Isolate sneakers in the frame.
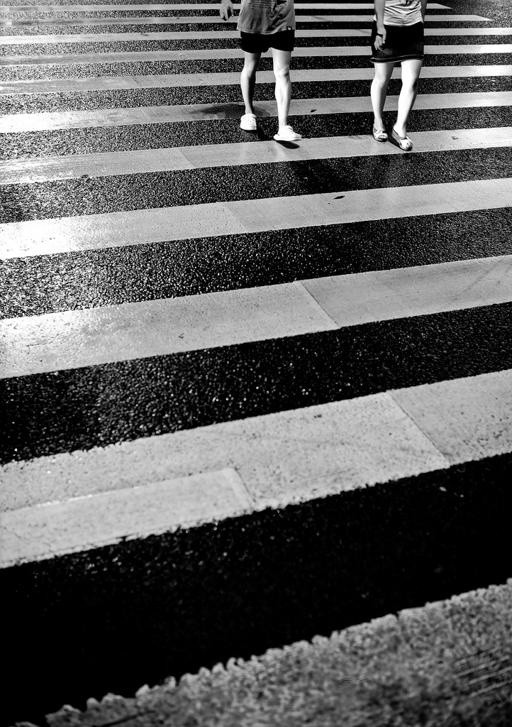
[392,129,413,150]
[373,127,389,141]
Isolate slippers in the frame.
[274,125,301,140]
[240,113,257,130]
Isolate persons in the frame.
[217,0,304,144]
[367,1,428,153]
